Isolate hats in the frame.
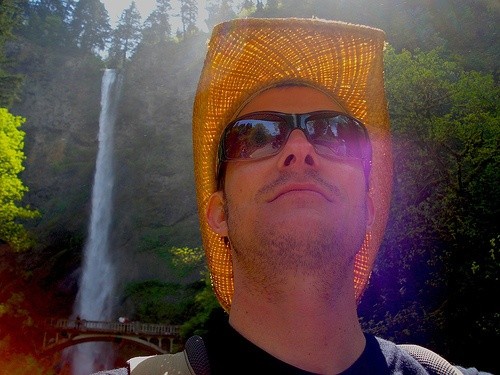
[192,17,393,314]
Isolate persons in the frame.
[89,80,492,375]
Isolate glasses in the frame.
[216,111,373,190]
